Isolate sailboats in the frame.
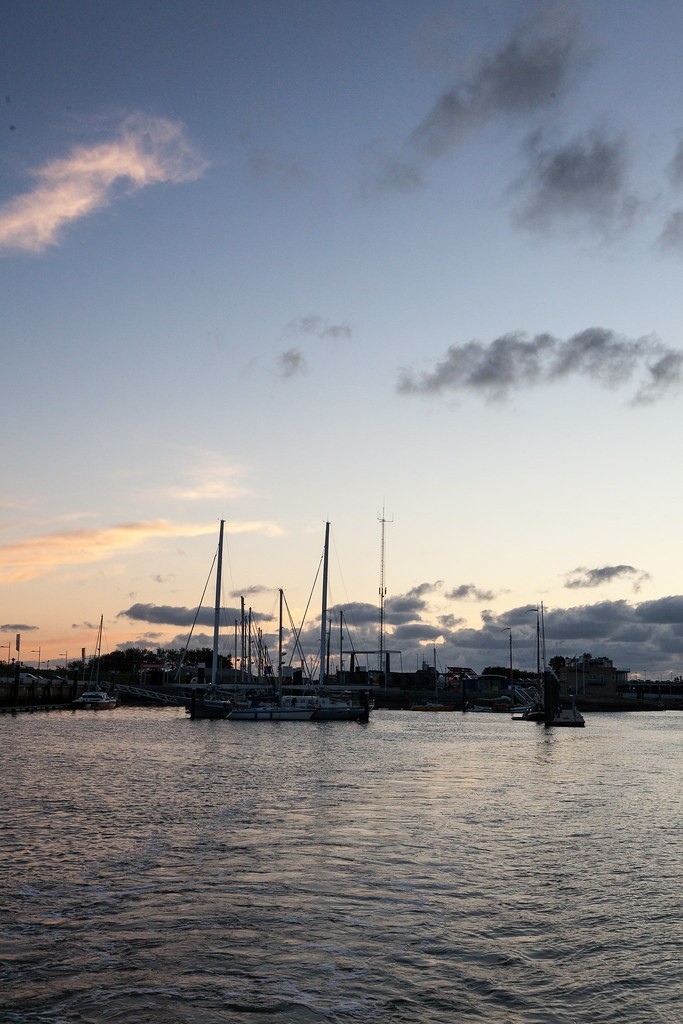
[70,614,117,710]
[170,520,389,722]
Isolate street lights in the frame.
[525,607,539,695]
[501,626,514,688]
[30,646,41,676]
[60,650,68,669]
[0,642,12,683]
